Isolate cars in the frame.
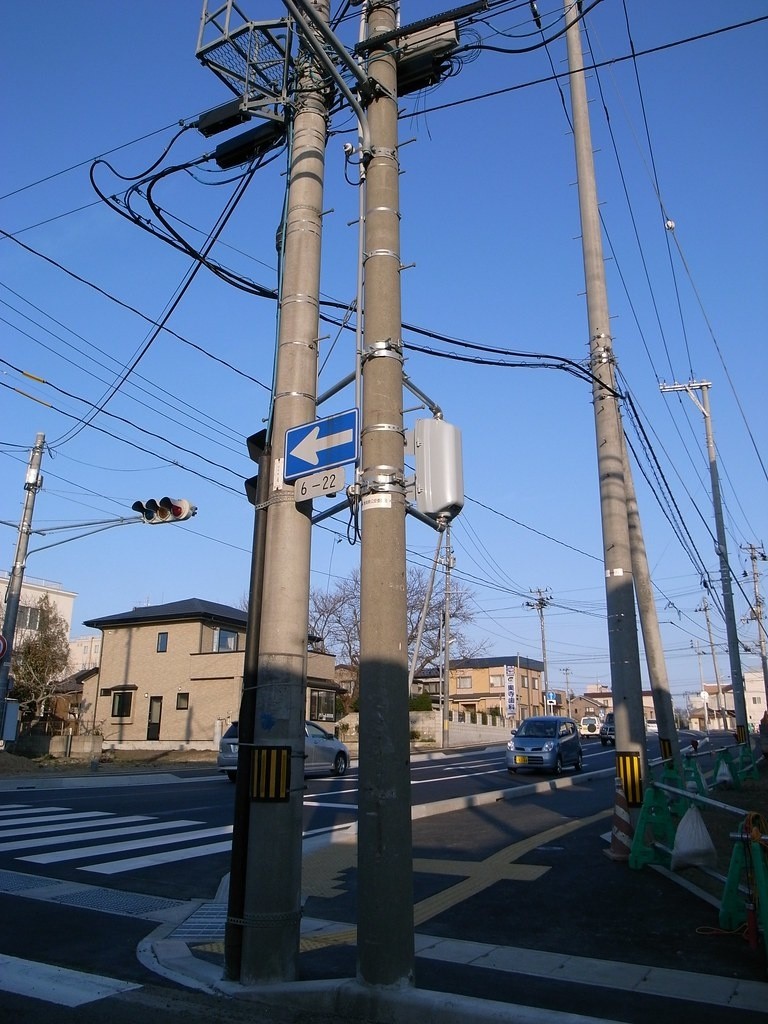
[214,716,351,786]
[504,715,584,777]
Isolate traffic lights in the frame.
[131,496,198,524]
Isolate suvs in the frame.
[600,712,615,746]
[578,716,604,739]
